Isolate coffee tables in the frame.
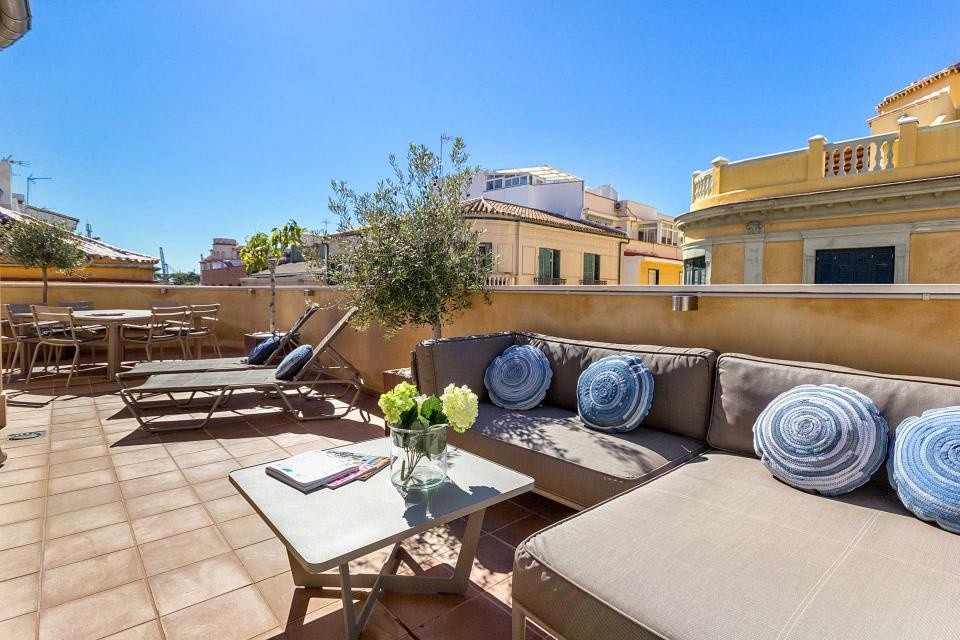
[229,434,536,640]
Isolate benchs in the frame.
[114,301,327,409]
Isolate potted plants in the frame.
[238,218,306,356]
[0,205,96,361]
[305,132,503,394]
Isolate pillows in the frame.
[751,383,890,499]
[886,405,960,535]
[577,353,655,433]
[484,344,554,412]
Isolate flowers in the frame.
[378,379,478,434]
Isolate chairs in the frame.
[119,303,371,434]
[0,299,224,396]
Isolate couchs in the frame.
[407,329,960,640]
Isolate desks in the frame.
[70,307,156,381]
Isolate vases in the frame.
[385,421,448,488]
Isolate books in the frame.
[265,449,391,493]
